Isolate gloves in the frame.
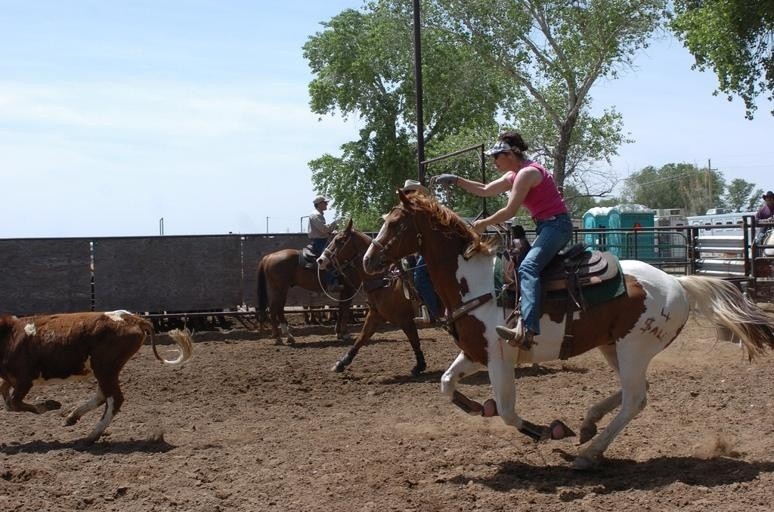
[430,174,458,184]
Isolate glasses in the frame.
[494,152,504,159]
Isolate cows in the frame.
[1,309,193,444]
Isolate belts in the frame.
[533,216,556,222]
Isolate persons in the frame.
[306,195,345,293]
[395,177,442,328]
[428,132,575,349]
[753,191,774,257]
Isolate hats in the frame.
[396,179,429,194]
[313,196,330,204]
[483,140,521,156]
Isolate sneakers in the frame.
[327,284,345,292]
[496,326,534,350]
[413,316,439,324]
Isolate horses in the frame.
[363,187,774,472]
[314,219,446,375]
[254,248,357,346]
